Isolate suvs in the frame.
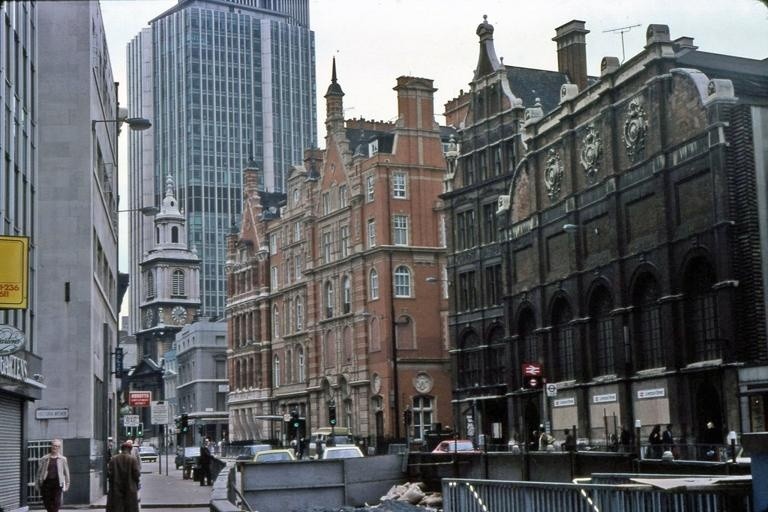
[236,445,272,460]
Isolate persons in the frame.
[562,428,576,451]
[609,432,618,452]
[292,434,335,460]
[200,438,214,487]
[508,426,547,451]
[707,423,738,447]
[105,440,144,512]
[649,423,675,456]
[38,439,70,512]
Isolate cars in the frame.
[138,446,157,463]
[316,444,365,460]
[254,449,295,462]
[176,447,201,469]
[431,438,483,454]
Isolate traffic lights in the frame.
[174,417,181,429]
[329,406,337,427]
[127,426,131,438]
[294,421,300,429]
[182,414,189,433]
[521,363,543,390]
[137,423,143,435]
[292,409,297,419]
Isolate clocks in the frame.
[171,306,188,323]
